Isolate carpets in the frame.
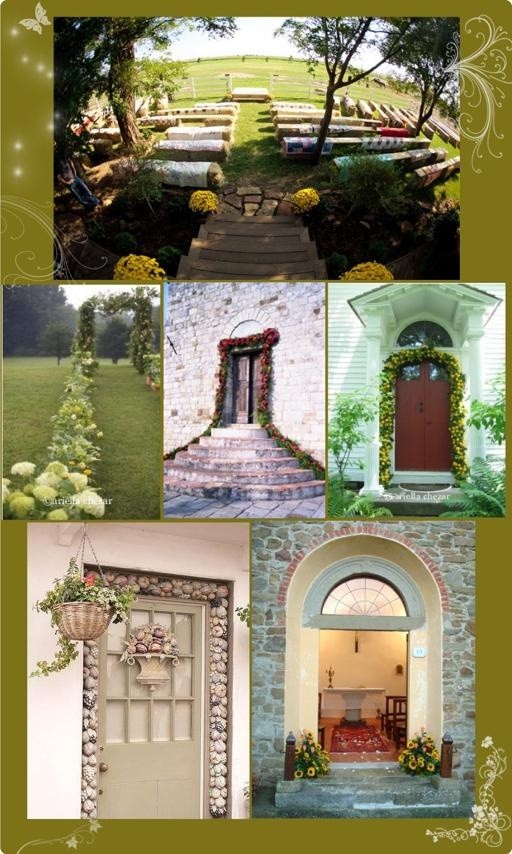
[328,723,391,753]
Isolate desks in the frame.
[322,686,386,728]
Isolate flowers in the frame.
[287,186,321,215]
[376,335,473,490]
[293,727,332,781]
[28,555,140,679]
[188,189,219,217]
[163,325,327,482]
[2,283,161,519]
[222,91,233,98]
[336,259,394,280]
[395,725,442,779]
[111,251,169,280]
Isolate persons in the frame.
[57,159,100,209]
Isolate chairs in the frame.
[318,692,327,750]
[380,694,407,752]
